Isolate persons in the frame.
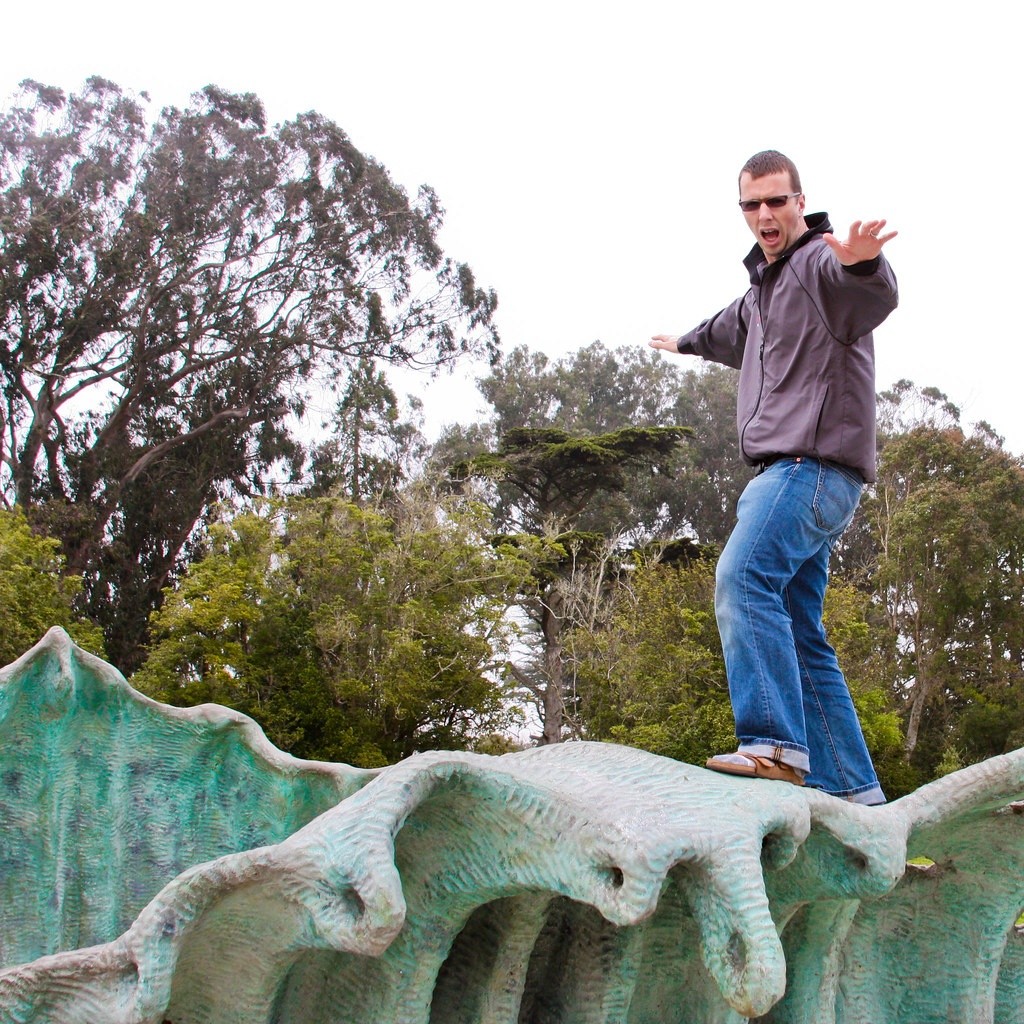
[648,150,900,806]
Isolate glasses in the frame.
[738,192,802,212]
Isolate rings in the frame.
[870,231,878,237]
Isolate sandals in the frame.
[705,752,805,785]
